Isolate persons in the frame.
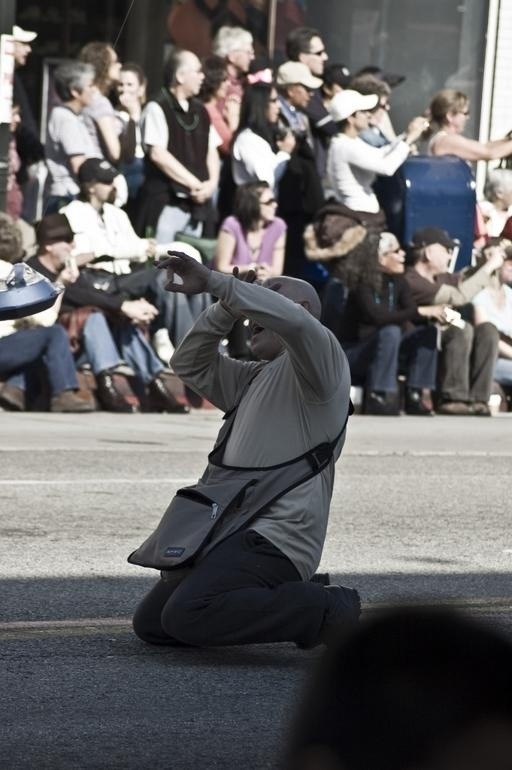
[0,23,512,417]
[133,249,360,653]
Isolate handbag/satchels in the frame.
[128,455,302,571]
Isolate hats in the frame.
[32,214,77,246]
[13,26,38,43]
[325,89,379,123]
[325,62,352,87]
[413,224,458,250]
[277,60,324,91]
[361,66,407,87]
[76,158,121,184]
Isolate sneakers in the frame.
[1,371,192,414]
[368,389,491,415]
[297,573,362,647]
